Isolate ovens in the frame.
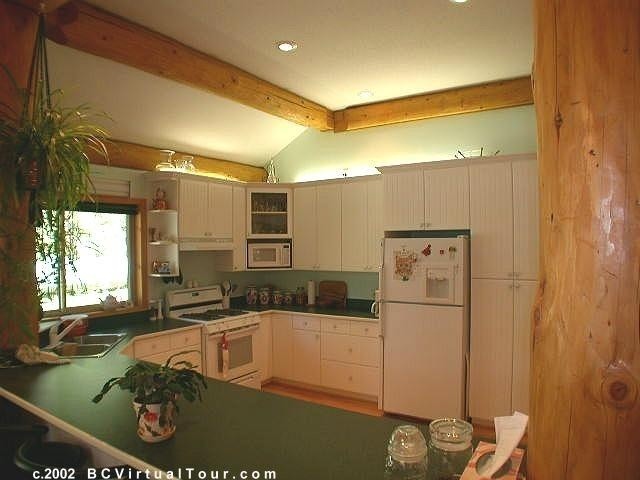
[204,314,262,384]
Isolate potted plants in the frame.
[0,13,121,364]
[91,349,207,443]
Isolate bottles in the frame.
[382,425,430,480]
[428,416,475,480]
[271,290,295,306]
[258,287,270,305]
[245,285,258,305]
[295,286,306,307]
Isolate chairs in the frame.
[0,422,148,479]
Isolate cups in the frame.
[222,296,230,308]
[120,300,126,305]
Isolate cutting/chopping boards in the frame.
[319,280,347,304]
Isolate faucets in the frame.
[49,316,84,343]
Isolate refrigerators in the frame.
[377,234,470,423]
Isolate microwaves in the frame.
[248,241,292,269]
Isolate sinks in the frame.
[65,333,127,345]
[45,343,111,358]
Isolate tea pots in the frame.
[98,294,117,305]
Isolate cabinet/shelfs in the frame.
[142,175,180,279]
[133,328,202,377]
[272,314,379,404]
[291,179,382,271]
[239,187,291,239]
[382,166,469,231]
[178,178,238,251]
[465,161,542,422]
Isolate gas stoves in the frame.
[177,307,250,322]
